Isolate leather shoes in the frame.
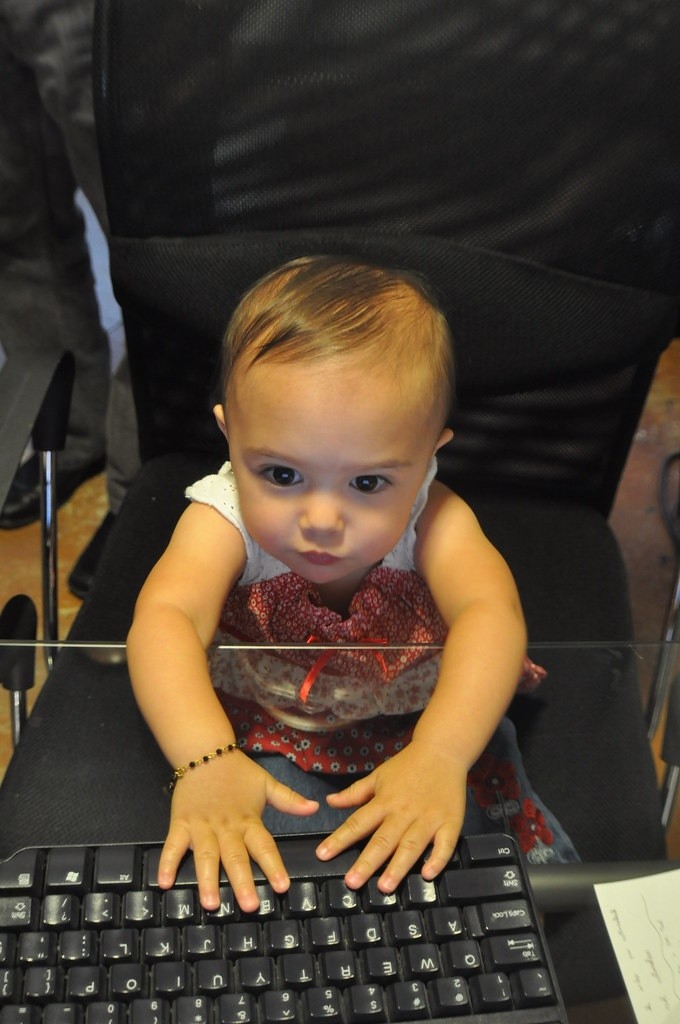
[0,455,105,529]
[68,511,115,599]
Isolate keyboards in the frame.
[0,832,571,1024]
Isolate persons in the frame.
[126,255,582,911]
[0,0,145,601]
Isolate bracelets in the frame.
[163,740,240,800]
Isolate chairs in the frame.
[0,0,680,861]
